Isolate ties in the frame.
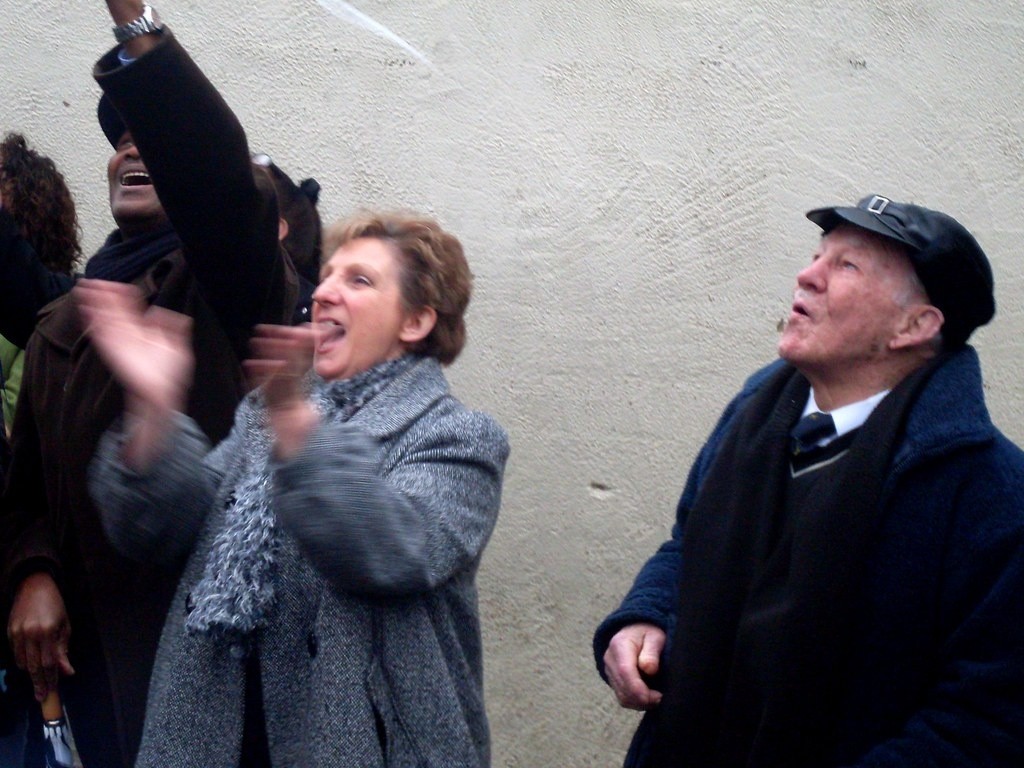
[790,412,835,457]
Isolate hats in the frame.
[97,92,131,153]
[806,193,995,360]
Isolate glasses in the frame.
[250,153,283,180]
[115,137,135,151]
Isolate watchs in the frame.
[111,5,163,45]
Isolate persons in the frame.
[0,0,327,768]
[593,198,1024,768]
[71,214,509,768]
